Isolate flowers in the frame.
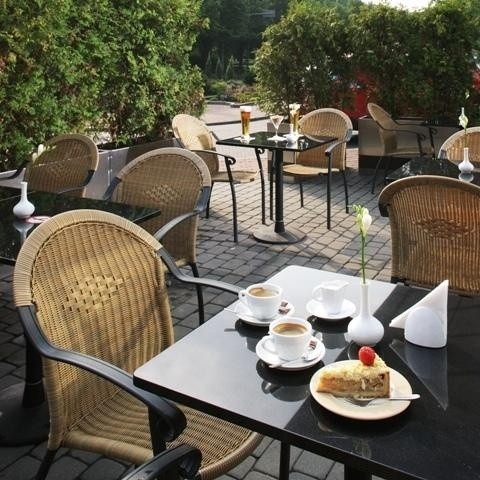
[344,198,375,284]
[288,102,301,124]
[25,133,58,181]
[454,104,471,147]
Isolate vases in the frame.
[11,217,35,248]
[11,180,37,219]
[346,283,385,348]
[459,147,476,174]
[458,171,475,183]
[286,123,299,144]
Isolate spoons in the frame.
[269,350,321,367]
[223,307,281,322]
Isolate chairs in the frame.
[119,437,205,480]
[364,99,443,196]
[166,110,269,243]
[99,144,216,333]
[0,130,97,201]
[7,203,294,480]
[373,171,480,298]
[436,126,479,161]
[266,105,356,230]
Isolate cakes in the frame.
[317,348,390,400]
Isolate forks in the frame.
[353,393,420,403]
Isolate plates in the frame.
[309,360,412,420]
[256,335,325,371]
[306,298,357,321]
[233,297,294,327]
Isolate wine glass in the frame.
[271,115,284,140]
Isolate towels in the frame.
[388,335,451,413]
[385,278,452,330]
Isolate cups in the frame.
[261,317,312,361]
[238,283,283,320]
[239,106,253,139]
[312,280,352,315]
